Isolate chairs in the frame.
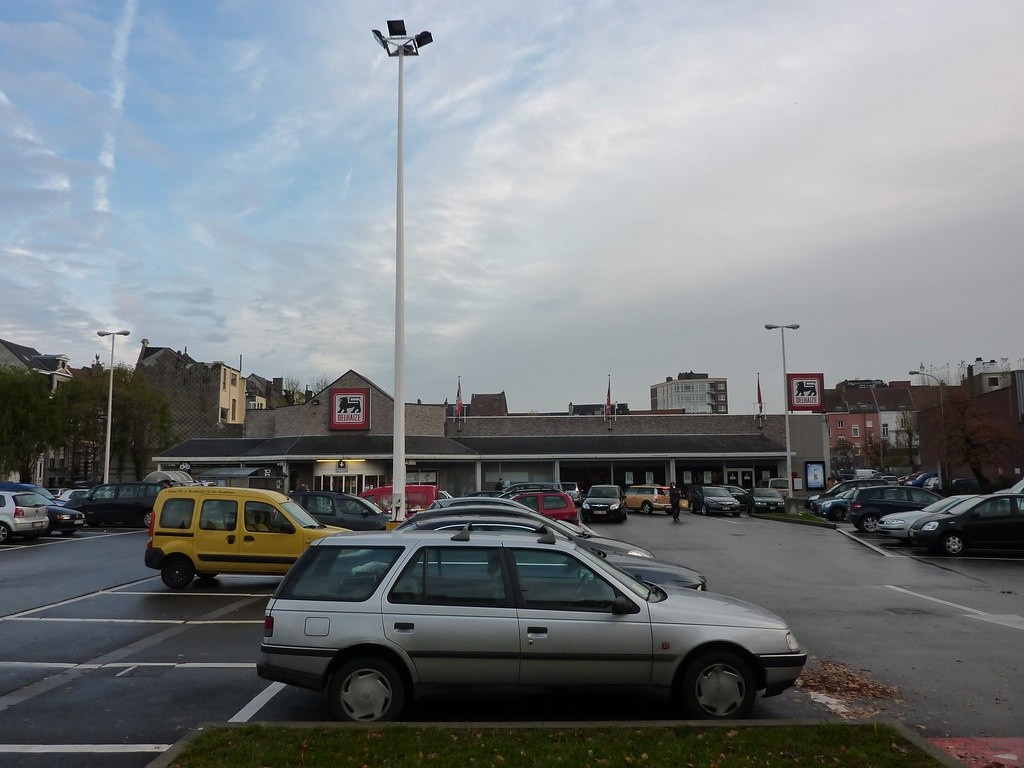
[246,511,269,532]
[120,487,131,499]
[986,501,999,515]
[310,497,319,512]
[486,557,525,605]
[893,490,906,500]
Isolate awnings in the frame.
[197,467,264,479]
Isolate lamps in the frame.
[312,399,320,404]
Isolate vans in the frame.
[63,483,163,528]
[806,479,946,534]
[145,484,439,589]
[758,479,790,498]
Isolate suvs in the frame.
[460,482,670,524]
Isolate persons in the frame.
[301,483,310,510]
[826,477,832,489]
[833,478,837,485]
[156,480,174,498]
[745,487,756,514]
[669,482,681,522]
[263,504,295,533]
[495,478,503,495]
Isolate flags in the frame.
[604,377,612,414]
[455,380,463,416]
[758,374,763,412]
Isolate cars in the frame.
[876,495,1024,556]
[688,485,785,517]
[0,483,85,545]
[257,497,806,721]
[880,471,980,494]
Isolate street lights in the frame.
[764,323,801,497]
[908,371,948,479]
[97,329,130,483]
[373,18,433,530]
